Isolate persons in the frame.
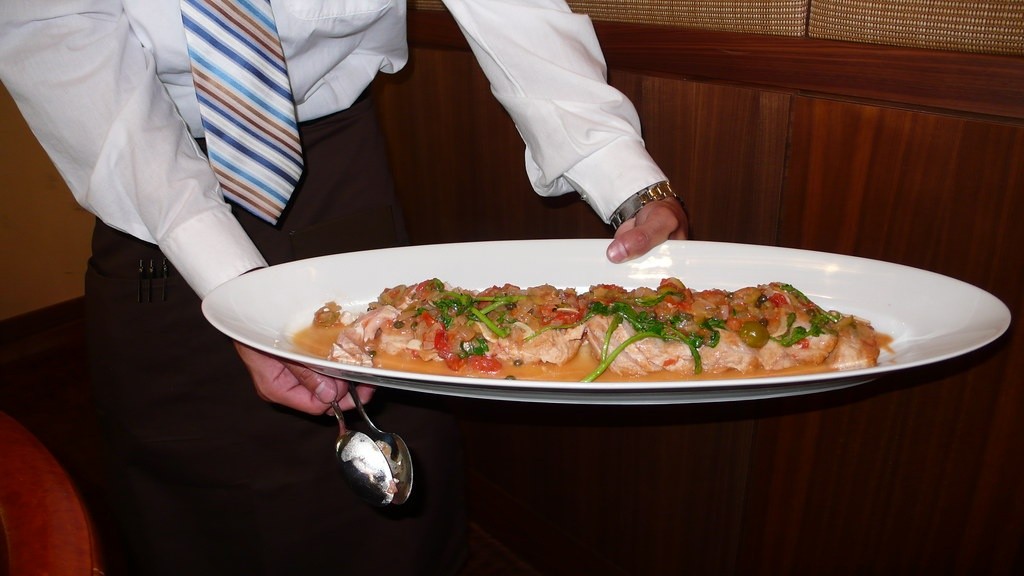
[0,0,687,576]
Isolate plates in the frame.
[201,237,1014,408]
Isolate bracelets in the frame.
[609,180,687,231]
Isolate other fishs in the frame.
[312,282,878,371]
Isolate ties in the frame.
[180,0,304,224]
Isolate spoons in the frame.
[329,400,395,507]
[344,383,414,509]
